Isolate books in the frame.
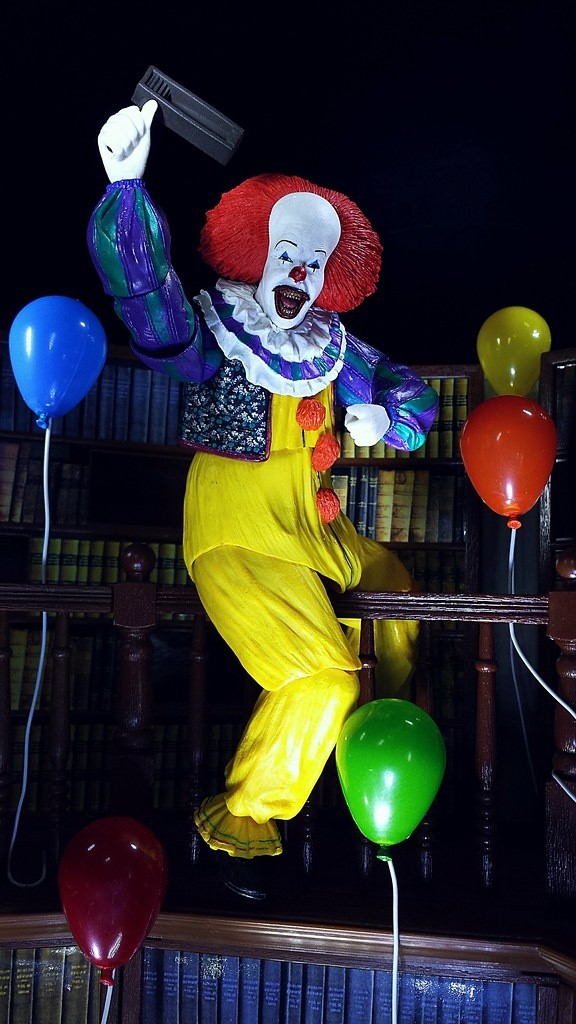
[0,443,465,543]
[25,537,210,621]
[9,628,244,813]
[432,665,457,735]
[0,350,469,459]
[0,943,575,1023]
[387,549,467,630]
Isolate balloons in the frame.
[475,304,553,400]
[59,816,161,985]
[10,295,107,426]
[334,700,441,860]
[460,396,557,527]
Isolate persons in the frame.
[85,100,440,921]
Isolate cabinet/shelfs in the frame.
[0,286,572,736]
